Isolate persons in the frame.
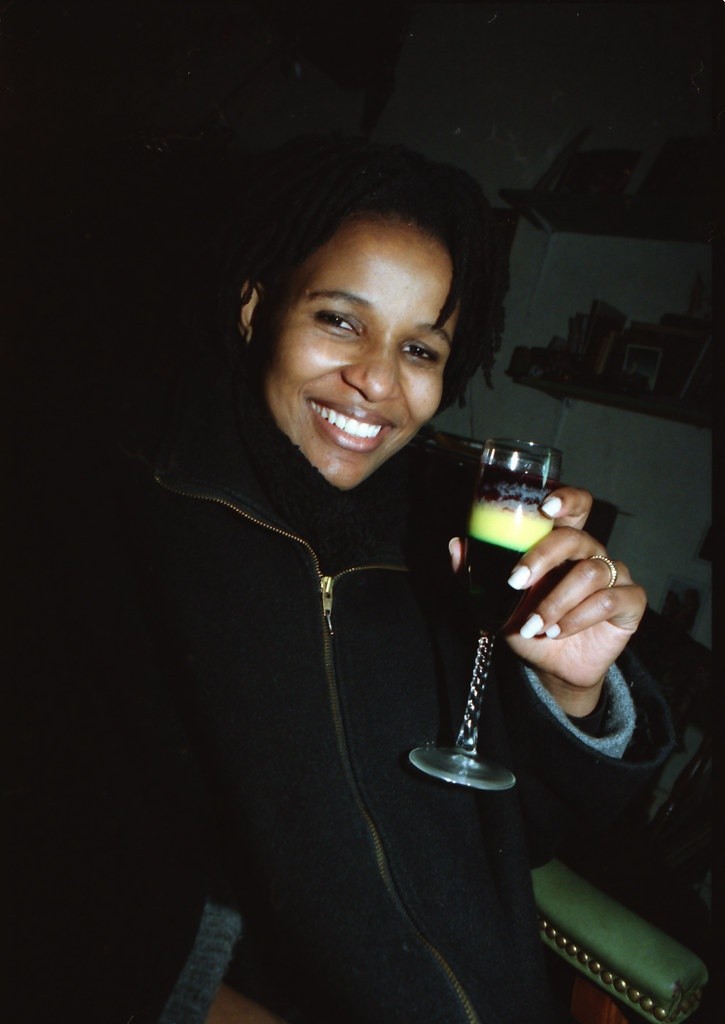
[0,143,646,1024]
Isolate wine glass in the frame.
[410,438,563,791]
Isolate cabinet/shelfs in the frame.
[498,184,713,428]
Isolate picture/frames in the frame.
[693,520,713,567]
[614,336,671,403]
[656,573,711,640]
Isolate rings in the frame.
[590,555,618,587]
